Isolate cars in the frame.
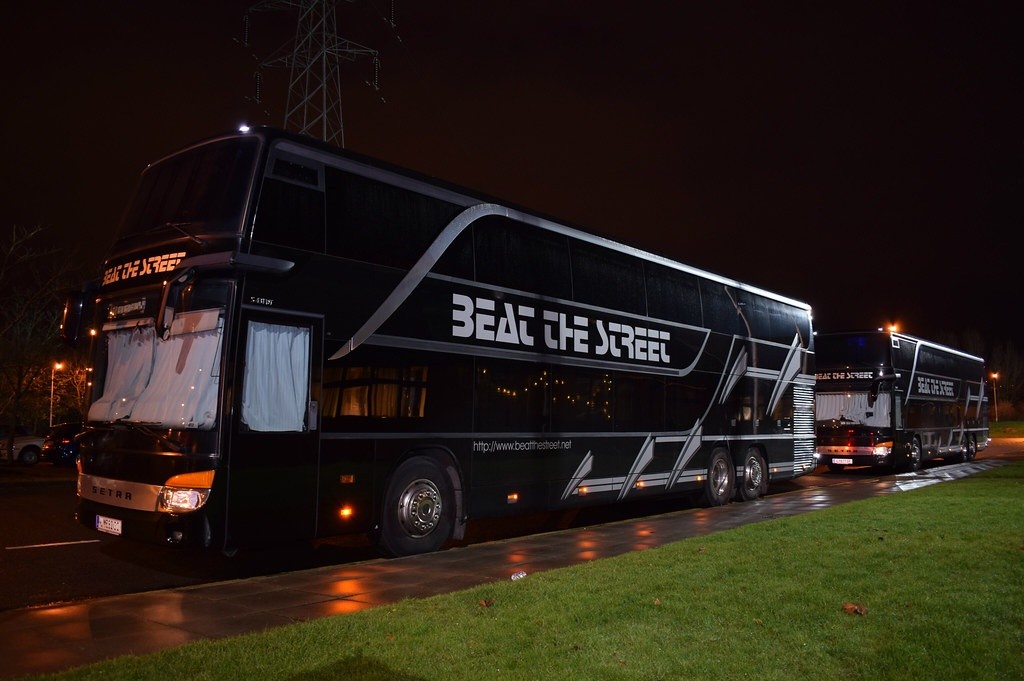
[39,418,84,474]
[0,433,49,467]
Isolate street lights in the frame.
[990,373,999,423]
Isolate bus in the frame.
[812,326,991,473]
[59,123,818,559]
